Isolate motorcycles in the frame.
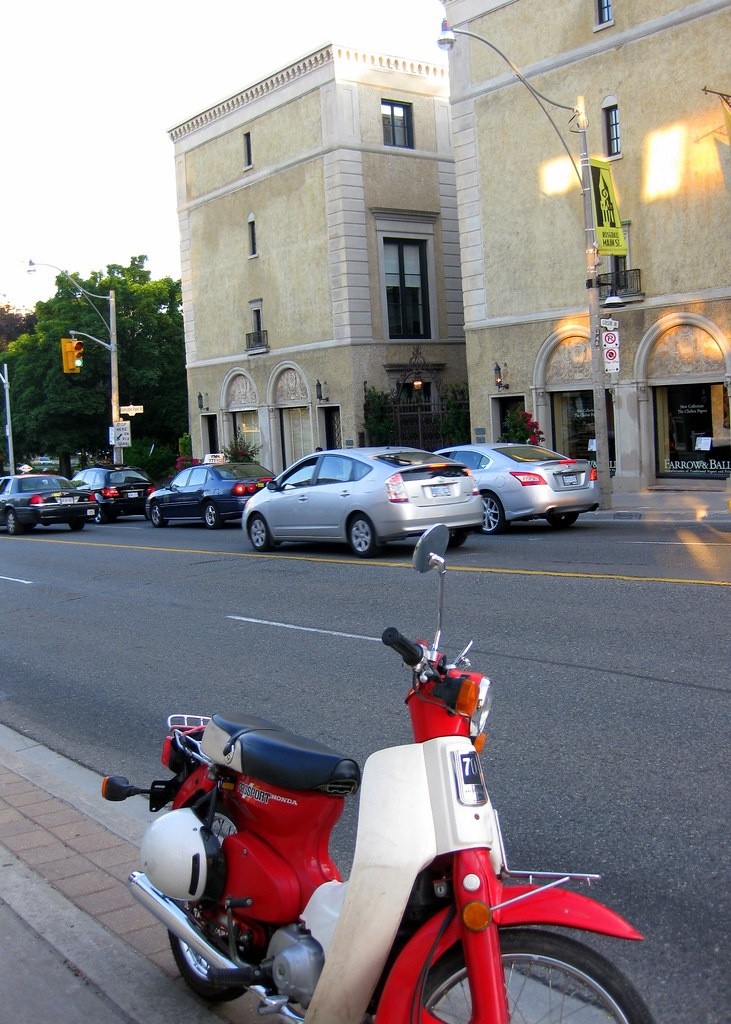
[100,521,660,1023]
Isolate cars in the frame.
[241,443,484,558]
[33,457,54,465]
[69,464,156,525]
[0,474,101,535]
[432,442,602,536]
[143,453,278,530]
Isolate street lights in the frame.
[436,20,618,508]
[391,370,424,446]
[26,259,126,465]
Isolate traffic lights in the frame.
[72,340,84,367]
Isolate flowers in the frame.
[174,457,198,470]
[499,402,546,446]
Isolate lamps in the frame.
[494,362,509,391]
[389,345,430,401]
